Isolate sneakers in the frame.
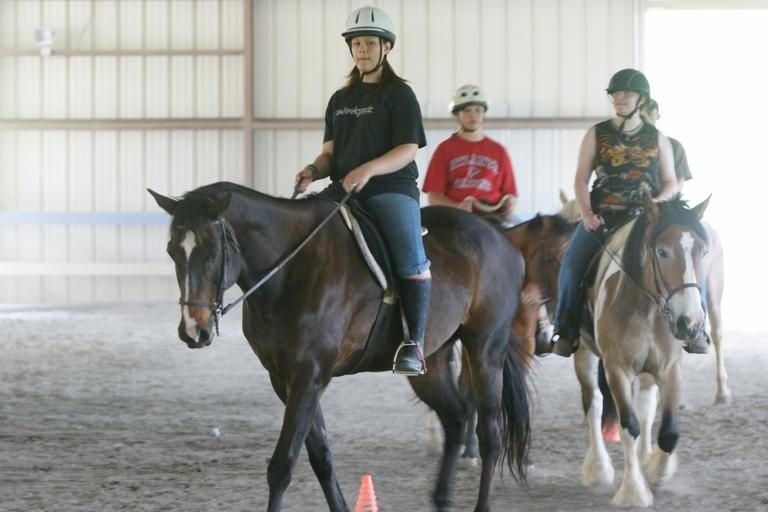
[552,337,575,357]
[686,334,710,355]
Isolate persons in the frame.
[640,98,693,198]
[423,85,518,223]
[294,7,432,373]
[551,69,712,357]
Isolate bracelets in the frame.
[306,164,319,180]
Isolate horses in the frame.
[569,187,714,509]
[454,208,574,467]
[553,186,731,444]
[144,181,536,512]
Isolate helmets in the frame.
[606,69,650,99]
[341,7,397,43]
[451,83,489,111]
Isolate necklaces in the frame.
[624,122,642,133]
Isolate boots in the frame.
[396,279,431,373]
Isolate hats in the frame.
[644,98,658,110]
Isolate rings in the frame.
[351,181,357,186]
